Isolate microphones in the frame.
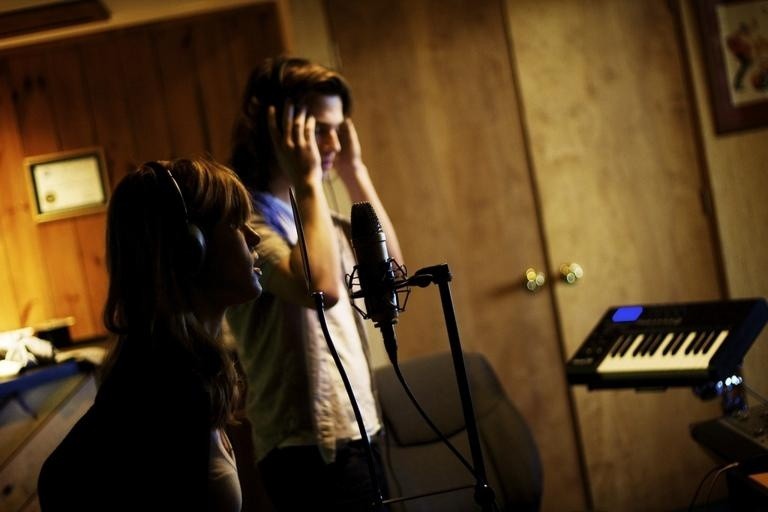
[350,199,400,325]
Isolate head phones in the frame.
[148,161,207,272]
[257,59,301,139]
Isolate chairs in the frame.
[370,348,545,511]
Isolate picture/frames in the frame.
[22,144,112,226]
[695,1,768,136]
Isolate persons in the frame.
[226,56,406,511]
[38,158,263,510]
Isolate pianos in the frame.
[564,299,768,389]
